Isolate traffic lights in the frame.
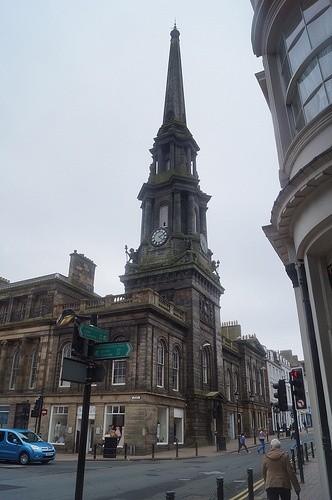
[60,356,88,386]
[272,378,288,412]
[291,367,308,409]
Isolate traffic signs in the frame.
[91,341,133,360]
[78,323,109,343]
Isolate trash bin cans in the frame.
[217,435,228,452]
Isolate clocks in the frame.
[200,233,208,255]
[150,228,170,247]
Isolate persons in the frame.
[107,427,117,436]
[238,434,251,454]
[9,435,17,441]
[263,439,300,500]
[39,432,48,442]
[55,421,64,443]
[27,435,34,440]
[292,429,295,438]
[258,429,266,453]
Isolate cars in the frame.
[0,428,56,464]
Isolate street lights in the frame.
[55,309,100,500]
